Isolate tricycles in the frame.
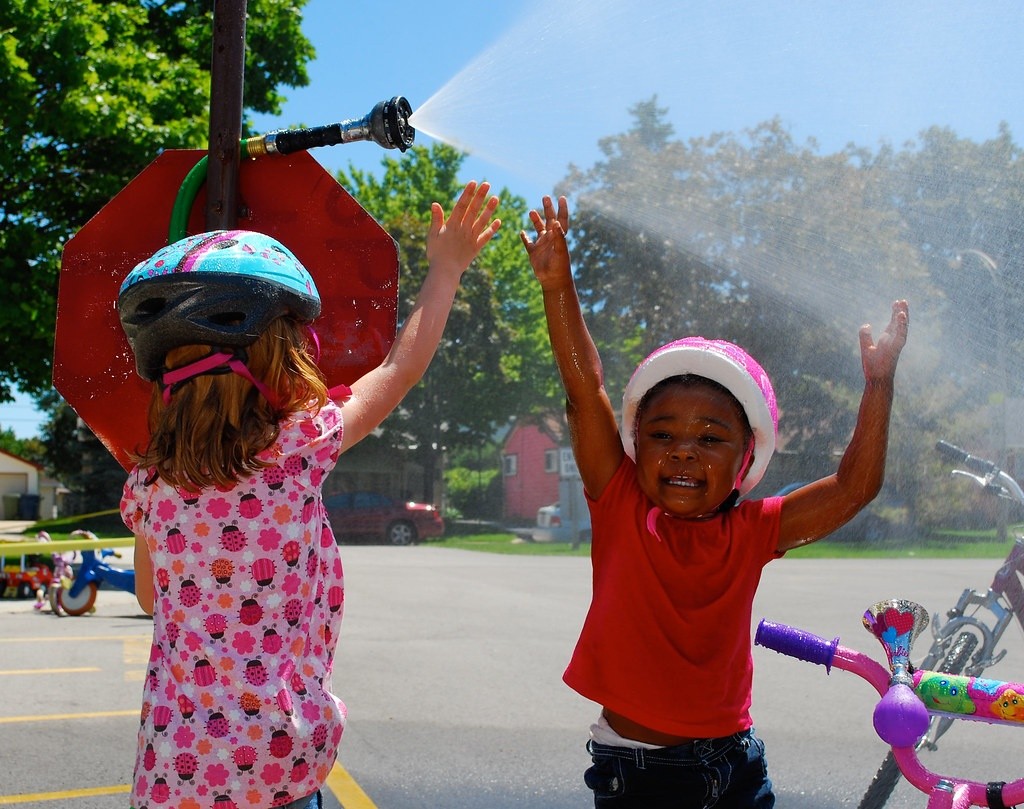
[39,528,144,616]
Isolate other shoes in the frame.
[34,602,44,610]
[57,604,63,610]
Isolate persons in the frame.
[33,560,52,611]
[37,529,52,541]
[117,178,502,809]
[53,556,74,581]
[79,526,98,539]
[521,194,910,809]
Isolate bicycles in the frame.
[754,595,1024,809]
[856,435,1024,808]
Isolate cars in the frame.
[0,538,53,599]
[534,502,562,531]
[322,487,445,545]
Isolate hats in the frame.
[53,556,66,565]
[622,335,780,501]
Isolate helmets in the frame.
[119,229,323,384]
